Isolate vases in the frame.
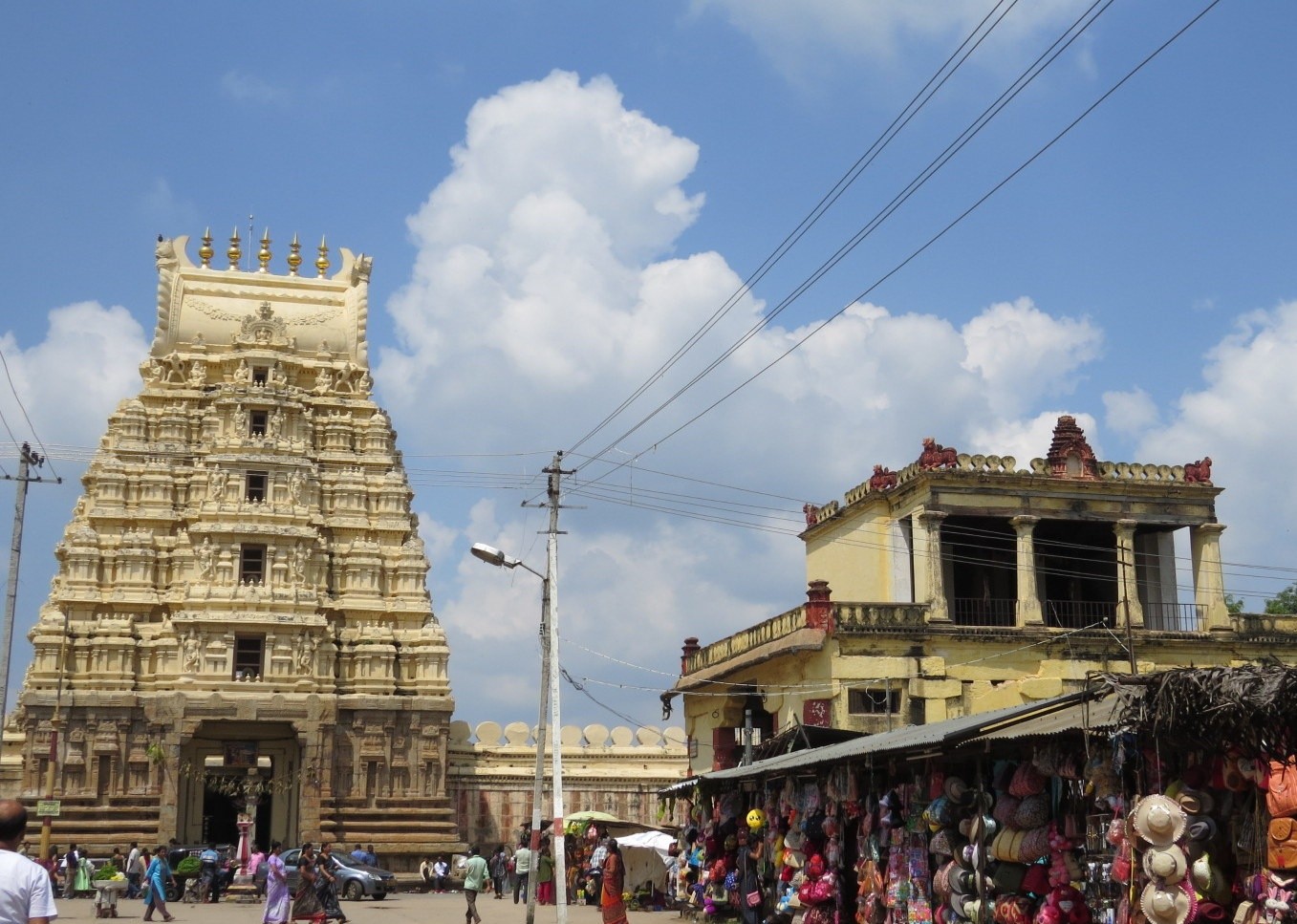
[92,880,129,918]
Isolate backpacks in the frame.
[1264,747,1297,817]
[1267,818,1297,871]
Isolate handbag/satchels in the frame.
[781,797,809,924]
[746,891,761,907]
[668,827,704,907]
[927,760,959,924]
[991,738,1079,924]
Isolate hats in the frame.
[1123,779,1231,924]
[942,775,998,924]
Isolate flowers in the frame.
[92,865,129,882]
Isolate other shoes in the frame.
[597,908,602,911]
[440,891,443,894]
[165,916,175,922]
[465,913,471,924]
[435,891,438,894]
[474,918,481,924]
[494,893,502,899]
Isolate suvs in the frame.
[160,843,238,903]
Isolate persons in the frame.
[0,799,58,924]
[352,843,380,869]
[736,832,764,924]
[18,838,223,922]
[249,841,349,924]
[460,845,492,923]
[488,832,629,924]
[420,856,452,894]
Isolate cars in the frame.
[252,847,397,901]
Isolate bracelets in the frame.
[328,875,331,879]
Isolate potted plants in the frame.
[577,889,586,906]
[621,892,632,908]
[177,856,203,903]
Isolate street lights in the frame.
[467,539,551,924]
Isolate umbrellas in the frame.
[564,809,616,821]
[613,830,679,896]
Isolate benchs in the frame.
[394,872,466,892]
